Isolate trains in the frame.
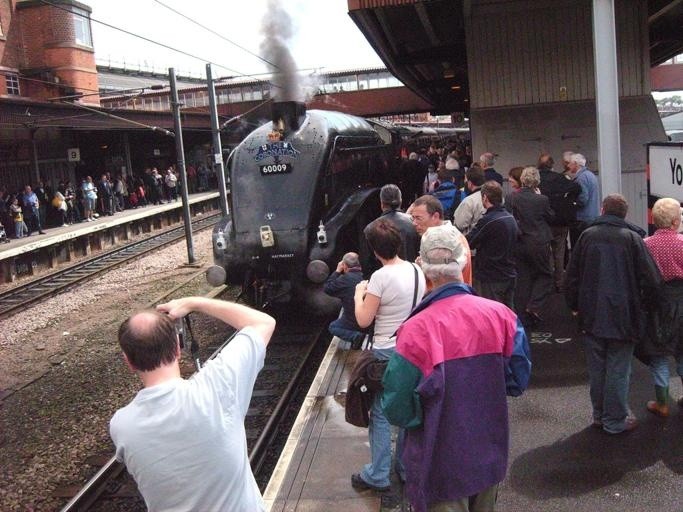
[206,97,471,311]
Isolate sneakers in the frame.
[591,412,638,434]
[351,331,366,350]
[16,193,180,240]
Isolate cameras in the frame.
[162,312,186,349]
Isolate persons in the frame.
[0,159,217,246]
[106,292,279,512]
[322,134,681,509]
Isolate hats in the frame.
[419,224,465,266]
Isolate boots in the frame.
[646,384,670,418]
[678,375,683,406]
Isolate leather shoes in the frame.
[525,306,545,322]
[350,470,392,493]
